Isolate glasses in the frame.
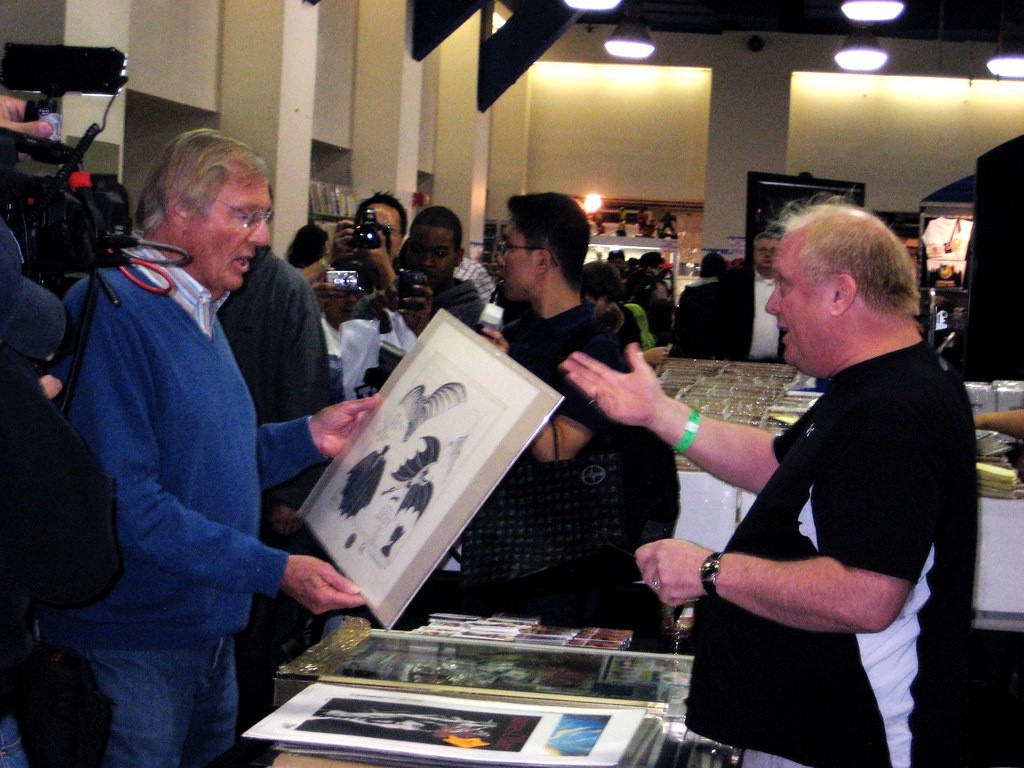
[496,239,559,267]
[213,198,273,227]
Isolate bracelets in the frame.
[672,408,701,454]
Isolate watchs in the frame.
[697,553,725,595]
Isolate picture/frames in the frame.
[297,308,565,633]
[745,170,866,274]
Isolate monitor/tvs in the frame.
[745,171,866,266]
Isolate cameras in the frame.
[342,207,392,254]
[398,269,427,309]
[326,271,362,292]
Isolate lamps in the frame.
[986,20,1024,76]
[605,19,656,60]
[834,34,887,72]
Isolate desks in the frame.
[678,471,1024,632]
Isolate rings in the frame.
[650,580,659,592]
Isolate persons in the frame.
[0,95,787,768]
[560,191,1024,768]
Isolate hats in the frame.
[641,252,676,271]
